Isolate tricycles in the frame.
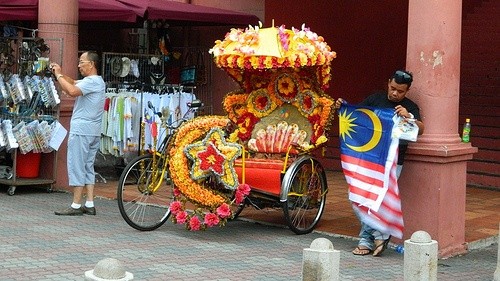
[118,19,336,235]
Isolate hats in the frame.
[111,56,123,76]
[129,60,140,78]
[118,57,131,77]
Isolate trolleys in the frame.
[0,72,61,197]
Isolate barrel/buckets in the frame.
[16,151,40,178]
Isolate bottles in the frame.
[463,118,471,143]
[387,242,406,255]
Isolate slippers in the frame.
[352,247,372,255]
[373,235,392,256]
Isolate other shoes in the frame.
[54,207,82,216]
[81,205,96,215]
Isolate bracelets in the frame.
[409,112,414,120]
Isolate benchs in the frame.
[233,120,306,198]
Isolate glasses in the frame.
[79,60,90,65]
[396,71,412,79]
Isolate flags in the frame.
[338,102,405,239]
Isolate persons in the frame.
[335,70,424,259]
[49,51,107,216]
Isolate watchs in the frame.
[56,74,64,81]
